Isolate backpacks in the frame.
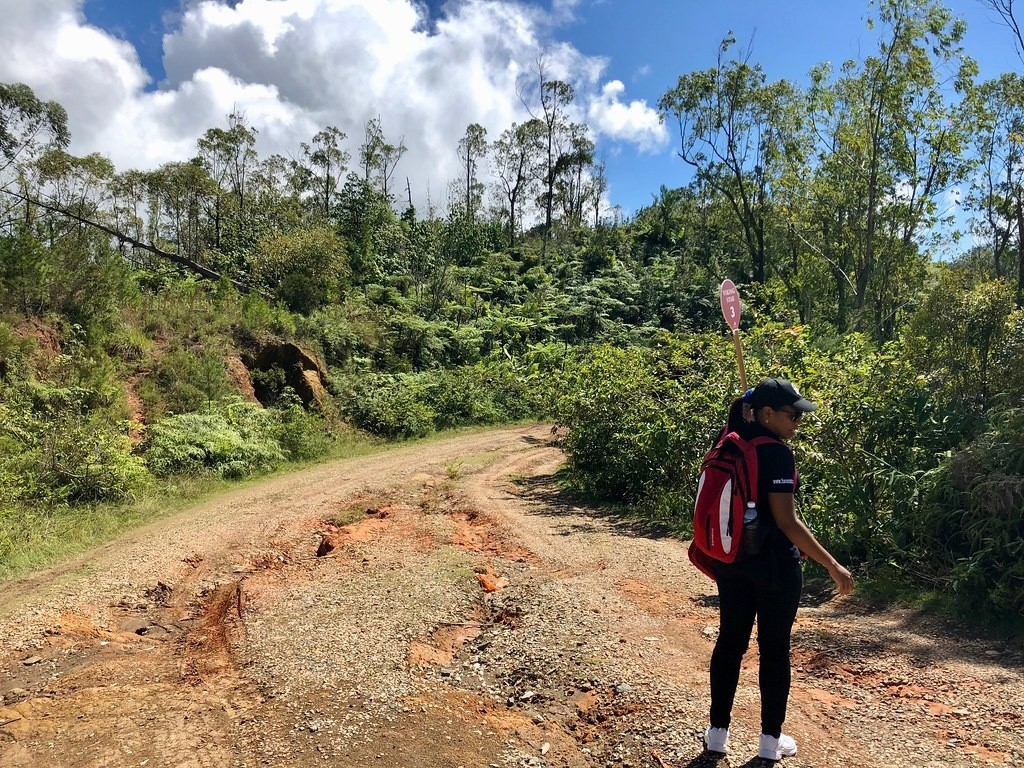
[693,432,797,564]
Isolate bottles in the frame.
[744,501,758,554]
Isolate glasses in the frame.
[771,408,803,422]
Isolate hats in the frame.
[750,378,819,412]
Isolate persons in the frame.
[693,377,856,760]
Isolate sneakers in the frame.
[758,733,797,761]
[704,726,729,753]
